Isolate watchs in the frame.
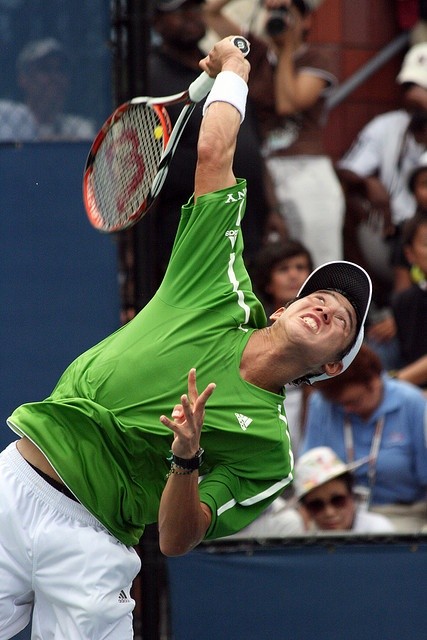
[171,445,206,469]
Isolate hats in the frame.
[296,260,372,383]
[270,446,376,517]
[395,40,427,84]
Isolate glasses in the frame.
[305,492,351,513]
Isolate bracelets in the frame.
[200,71,248,126]
[163,457,195,480]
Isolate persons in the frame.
[292,343,426,536]
[361,150,426,371]
[268,444,391,538]
[242,0,348,271]
[0,34,373,640]
[331,40,426,276]
[0,33,97,144]
[389,213,426,396]
[246,238,314,328]
